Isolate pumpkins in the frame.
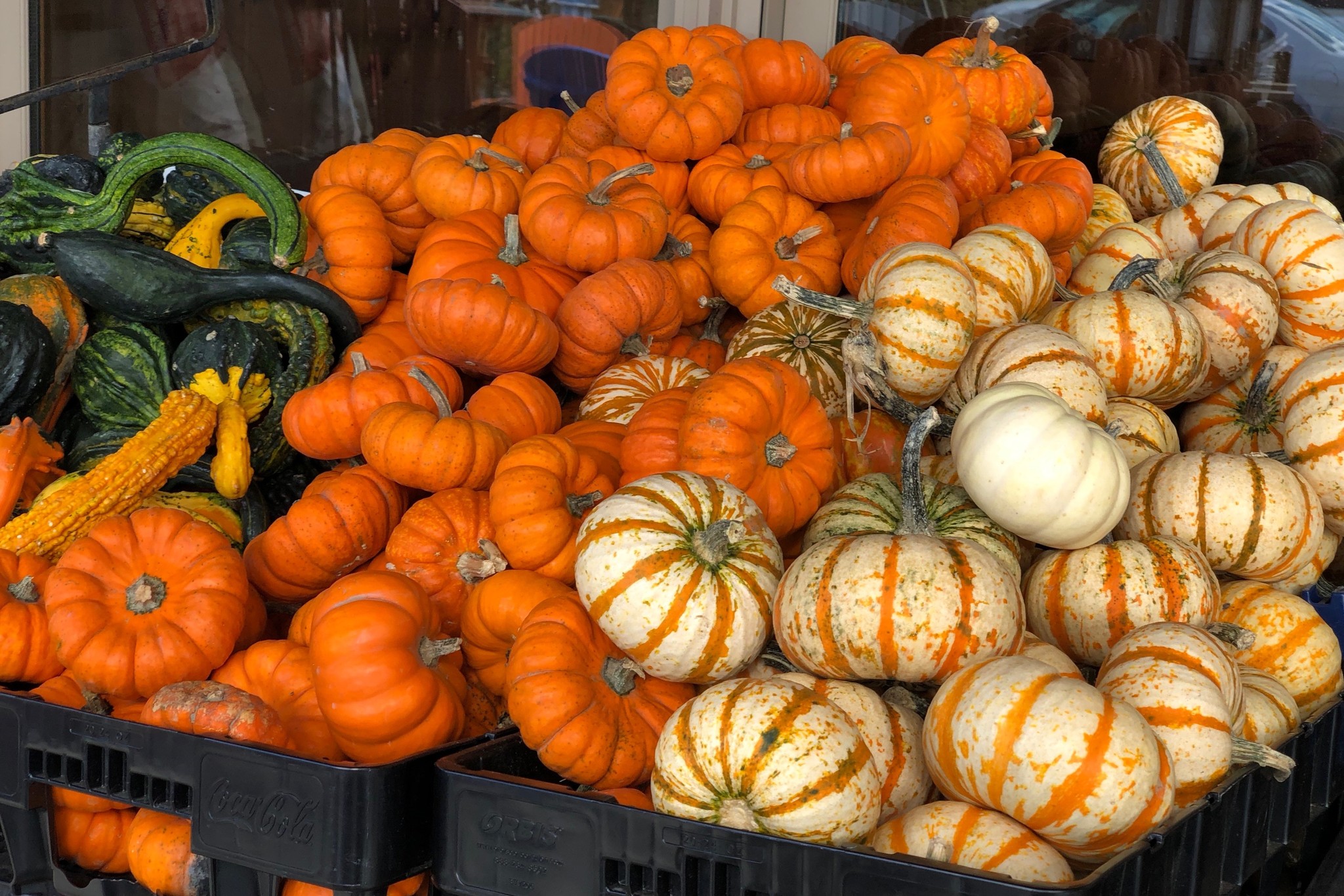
[0,14,1343,896]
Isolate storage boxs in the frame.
[0,680,1343,896]
